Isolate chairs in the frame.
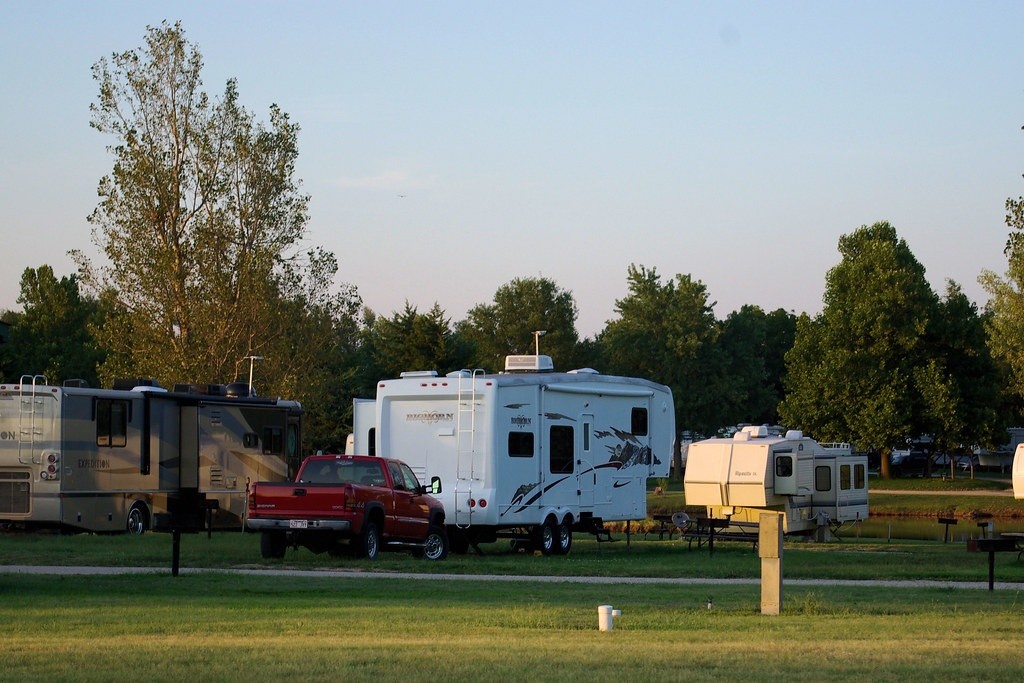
[317,472,340,483]
[360,475,382,486]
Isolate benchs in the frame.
[1000,532,1024,560]
[681,518,760,554]
